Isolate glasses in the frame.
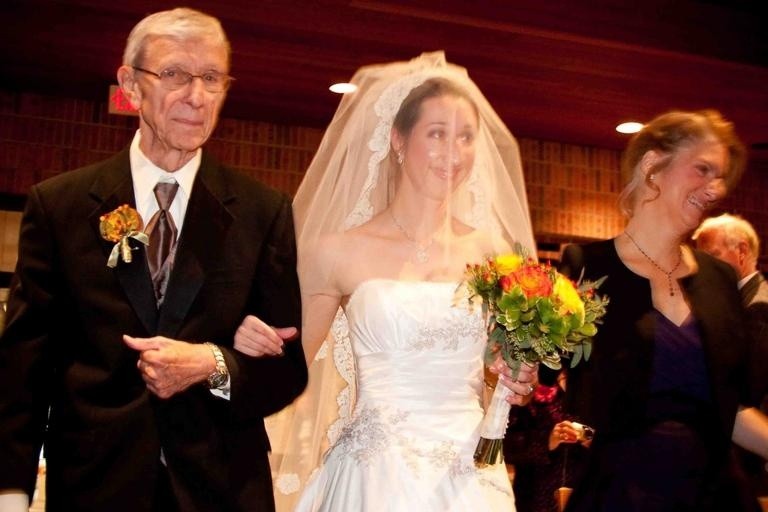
[131,65,238,94]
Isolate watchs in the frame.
[206,342,228,390]
[583,423,593,444]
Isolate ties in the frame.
[143,181,180,310]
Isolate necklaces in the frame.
[387,207,451,263]
[624,231,683,296]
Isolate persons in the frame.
[504,109,767,510]
[695,215,766,460]
[506,394,596,511]
[2,8,309,510]
[234,50,540,511]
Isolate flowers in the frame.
[449,245,610,468]
[99,203,149,267]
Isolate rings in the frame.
[529,385,535,395]
[560,433,563,441]
